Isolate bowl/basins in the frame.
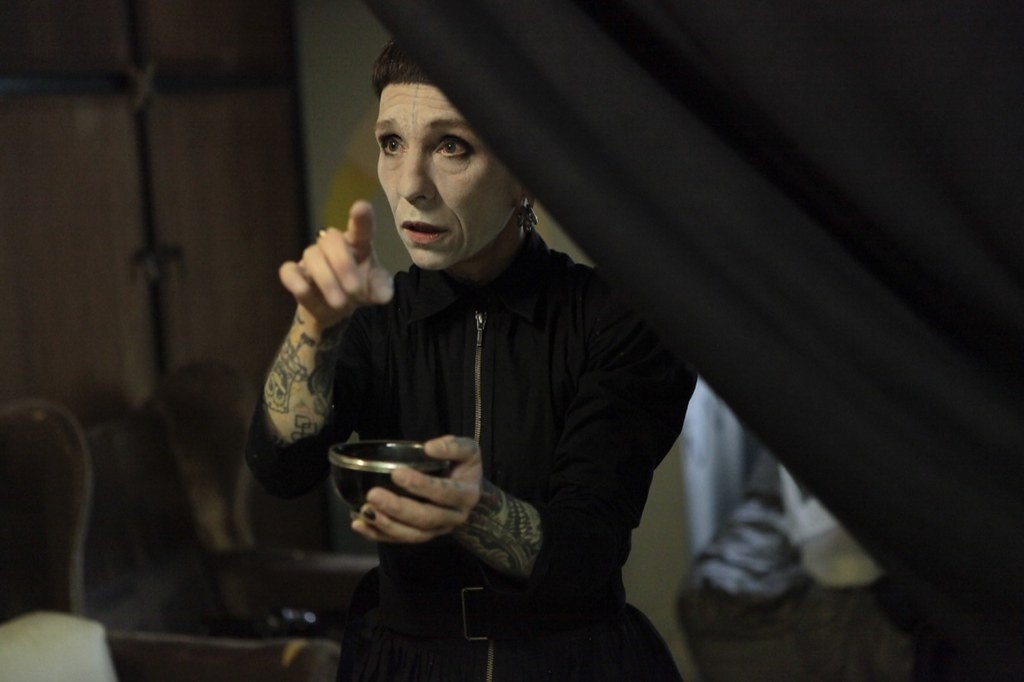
[327,442,454,514]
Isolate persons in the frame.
[678,379,913,682]
[245,35,698,682]
